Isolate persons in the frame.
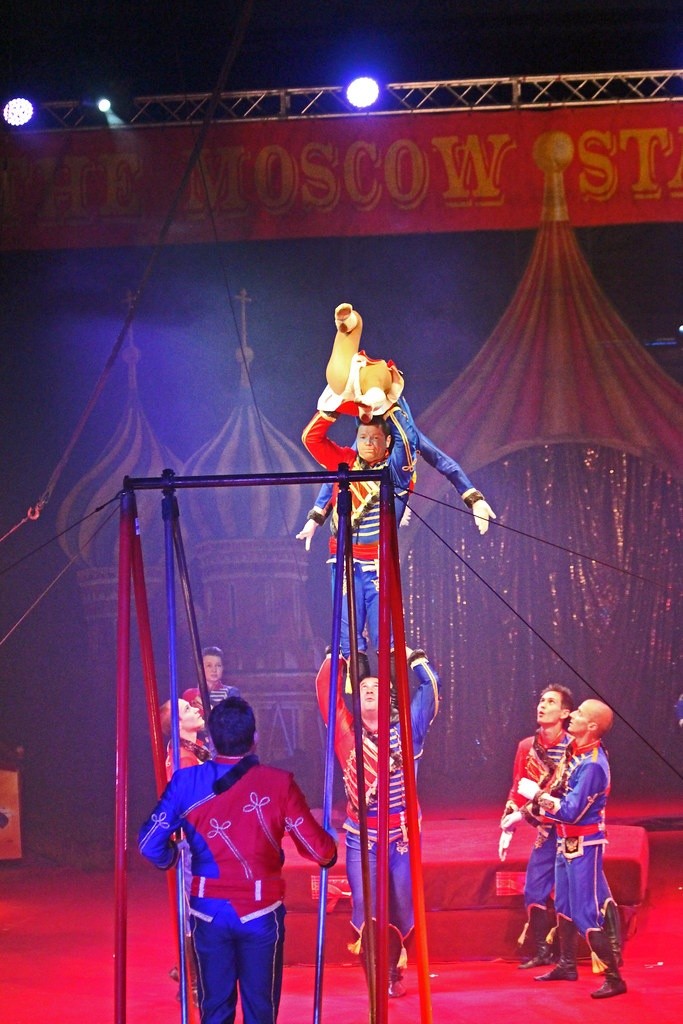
[137,696,337,1024]
[159,646,241,1009]
[296,303,497,714]
[315,645,443,998]
[499,685,627,999]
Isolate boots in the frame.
[585,927,627,999]
[375,648,402,716]
[346,916,415,998]
[168,931,202,1008]
[600,897,624,967]
[533,911,578,982]
[517,902,562,970]
[342,651,372,697]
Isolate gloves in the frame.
[460,487,497,535]
[295,504,326,552]
[500,810,525,830]
[497,830,514,862]
[516,776,544,801]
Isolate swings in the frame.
[160,481,374,1024]
